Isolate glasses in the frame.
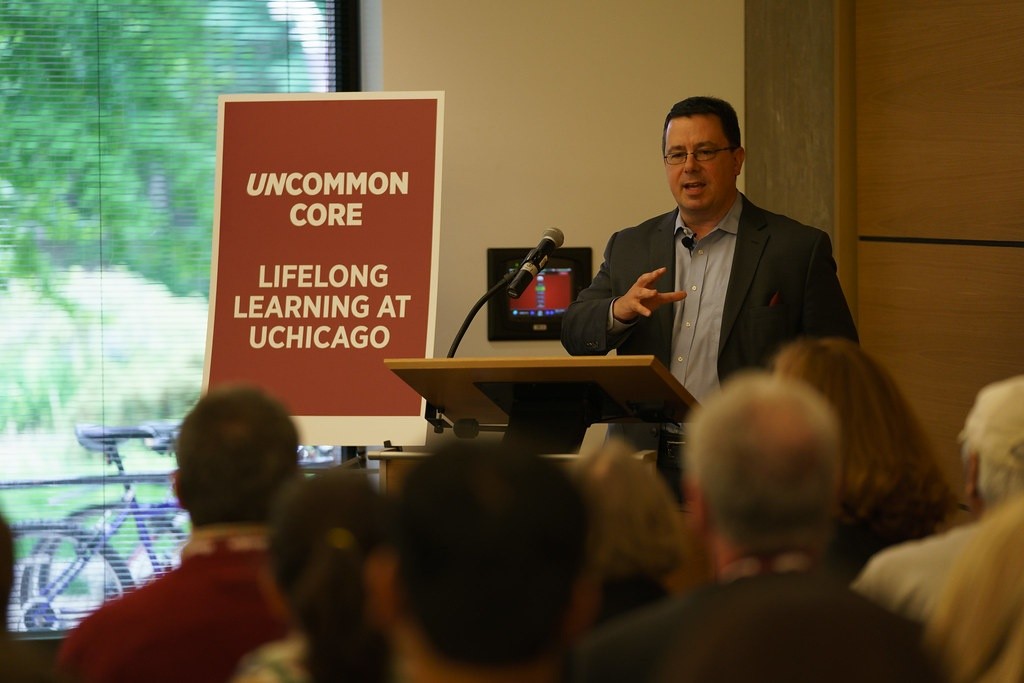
[665,146,738,165]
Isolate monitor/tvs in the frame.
[487,247,592,341]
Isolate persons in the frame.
[242,338,1024,683]
[563,98,859,406]
[47,383,306,683]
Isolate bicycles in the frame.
[8,418,340,632]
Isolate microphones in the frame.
[507,227,565,300]
[681,236,694,249]
